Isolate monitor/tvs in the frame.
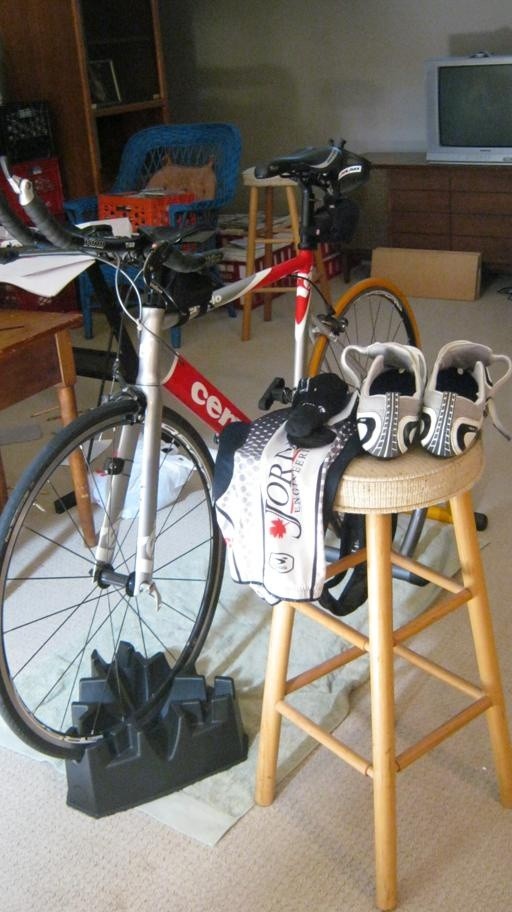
[425,56,512,166]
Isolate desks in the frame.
[0,308,97,555]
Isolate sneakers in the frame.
[328,341,428,461]
[421,338,512,458]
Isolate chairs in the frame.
[66,122,243,350]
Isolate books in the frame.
[116,187,297,262]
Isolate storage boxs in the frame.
[368,244,484,303]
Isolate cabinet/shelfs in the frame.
[359,152,511,276]
[2,0,171,203]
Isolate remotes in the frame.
[474,50,494,58]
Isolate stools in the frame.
[256,440,511,911]
[240,169,333,341]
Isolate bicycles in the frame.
[0,146,425,762]
